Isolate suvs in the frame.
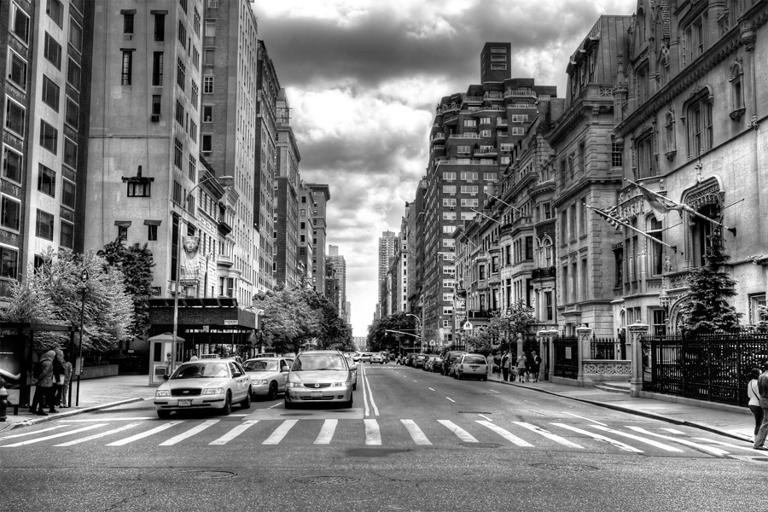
[448,353,488,381]
[440,350,466,375]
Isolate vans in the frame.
[353,352,374,363]
[380,351,390,362]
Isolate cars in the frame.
[344,354,357,391]
[242,356,294,401]
[283,348,352,409]
[404,352,443,372]
[153,358,253,420]
[370,354,383,364]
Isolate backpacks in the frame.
[33,358,49,378]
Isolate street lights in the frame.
[405,313,421,330]
[73,269,89,406]
[167,174,234,376]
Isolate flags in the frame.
[472,210,486,225]
[590,206,630,232]
[484,195,501,209]
[637,185,685,222]
[459,233,469,245]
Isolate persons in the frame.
[486,349,542,383]
[747,367,762,441]
[28,348,72,414]
[181,236,201,278]
[753,360,768,450]
[190,353,198,361]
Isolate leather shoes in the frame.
[755,444,767,450]
[49,408,58,412]
[37,410,47,416]
[29,408,37,414]
